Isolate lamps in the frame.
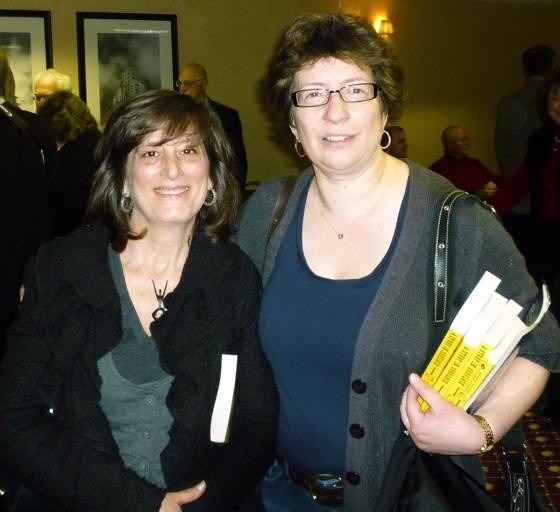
[379,19,395,42]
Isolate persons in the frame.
[491,73,560,323]
[428,124,506,203]
[228,10,560,510]
[382,126,409,159]
[0,86,284,511]
[0,67,105,344]
[494,44,559,217]
[176,62,248,196]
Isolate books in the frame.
[206,353,241,447]
[418,270,530,413]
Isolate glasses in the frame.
[291,83,382,107]
[175,79,203,87]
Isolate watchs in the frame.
[470,413,496,458]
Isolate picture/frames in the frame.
[75,11,178,133]
[0,9,55,118]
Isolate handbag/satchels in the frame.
[375,435,550,512]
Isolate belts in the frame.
[274,448,344,507]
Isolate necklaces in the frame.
[317,200,361,240]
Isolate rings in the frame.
[404,430,410,437]
